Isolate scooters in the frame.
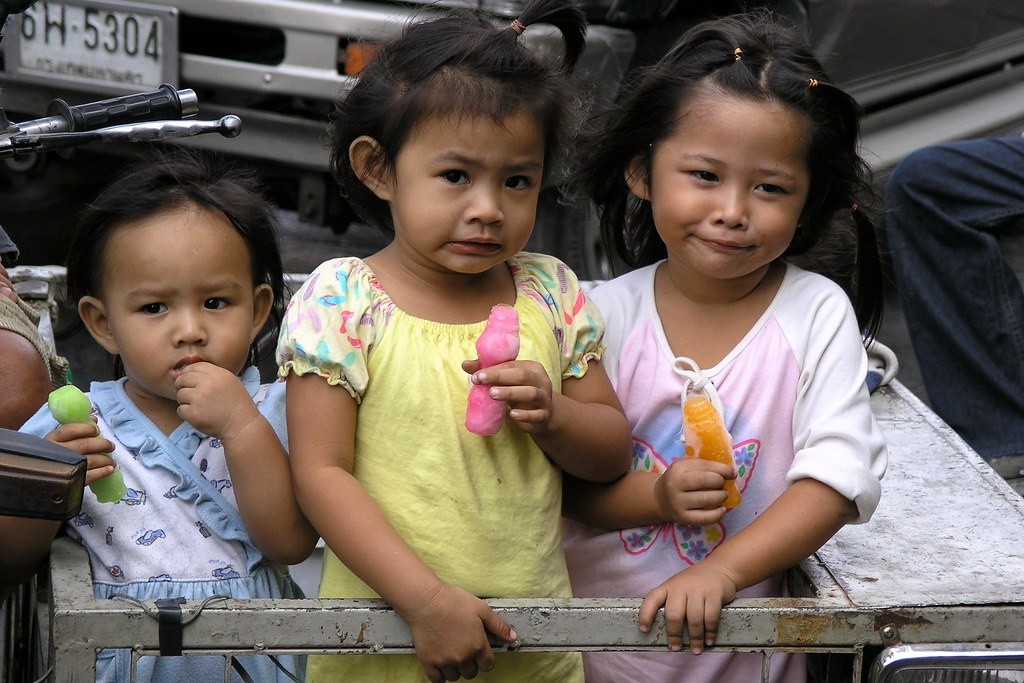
[1,81,1024,683]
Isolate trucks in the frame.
[0,0,1023,286]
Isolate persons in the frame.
[561,10,889,683]
[0,257,61,434]
[0,161,321,683]
[883,136,1024,460]
[276,1,633,683]
